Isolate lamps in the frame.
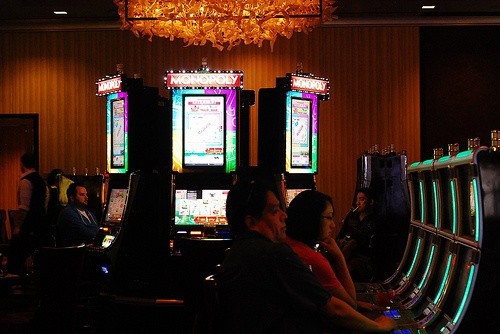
[111,0,338,52]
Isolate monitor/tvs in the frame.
[176,189,231,230]
[380,147,483,334]
[105,188,129,224]
[171,87,240,176]
[283,91,320,173]
[285,188,312,209]
[106,93,130,174]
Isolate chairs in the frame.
[0,208,33,277]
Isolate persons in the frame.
[337,188,390,282]
[57,182,100,245]
[281,191,359,311]
[48,168,66,217]
[215,180,396,333]
[11,154,50,274]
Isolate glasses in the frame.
[321,215,334,220]
